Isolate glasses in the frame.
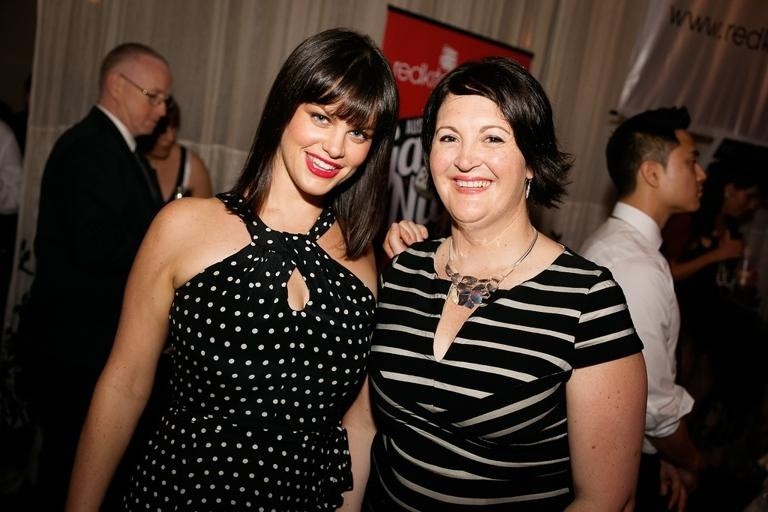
[122,75,173,107]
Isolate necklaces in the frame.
[445,225,538,310]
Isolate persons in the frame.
[577,104,767,512]
[0,41,214,512]
[68,28,429,512]
[341,56,648,512]
